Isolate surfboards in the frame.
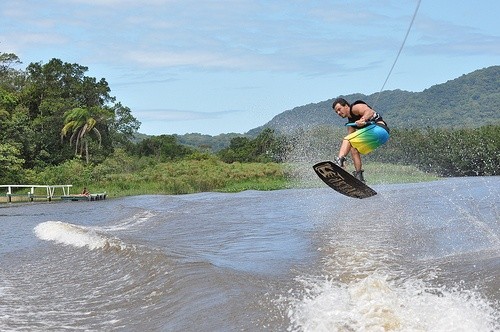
[313,161,377,199]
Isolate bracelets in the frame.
[361,117,366,120]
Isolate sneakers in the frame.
[332,155,348,170]
[352,169,366,183]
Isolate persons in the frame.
[333,97,389,184]
[83,187,89,196]
